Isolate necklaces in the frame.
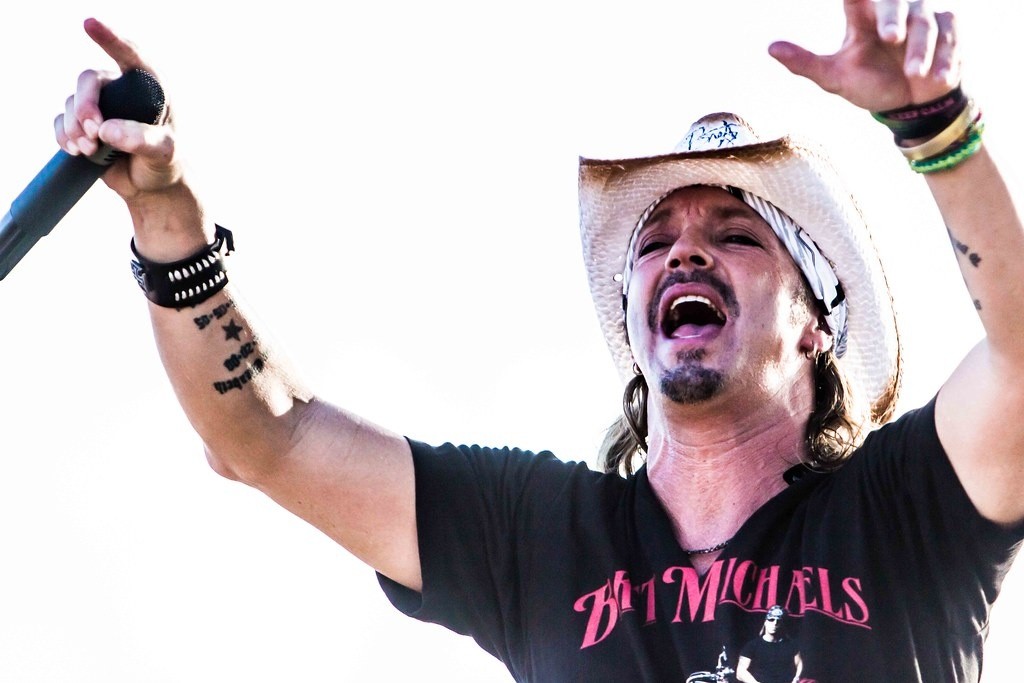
[685,540,730,557]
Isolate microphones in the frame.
[0,66,165,282]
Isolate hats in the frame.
[576,113,904,423]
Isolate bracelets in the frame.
[870,81,986,174]
[130,223,235,309]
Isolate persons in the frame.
[736,605,803,683]
[55,0,1024,683]
